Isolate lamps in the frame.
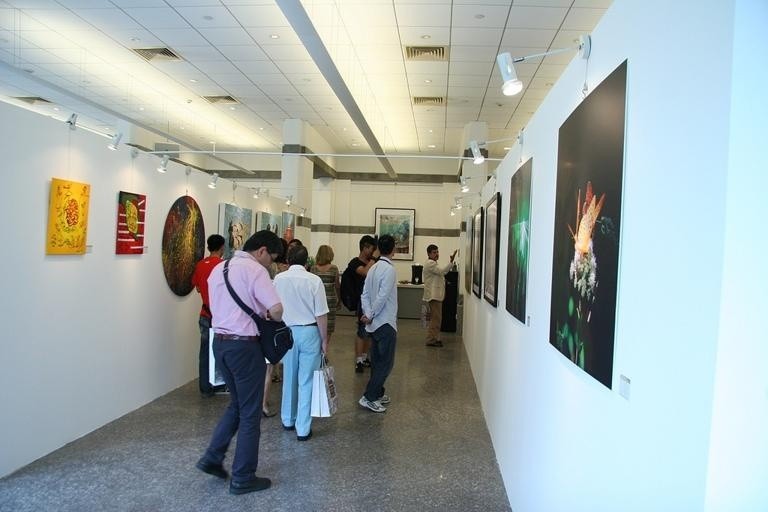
[449,176,495,218]
[496,33,590,100]
[470,127,523,167]
[129,148,172,175]
[66,113,124,150]
[185,163,311,219]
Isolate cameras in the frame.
[371,235,378,257]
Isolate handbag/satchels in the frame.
[256,316,294,364]
[309,352,338,419]
[208,326,226,386]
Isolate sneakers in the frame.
[359,390,391,413]
[201,387,230,399]
[355,356,372,373]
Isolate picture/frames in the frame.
[483,191,503,308]
[372,207,416,263]
[472,206,483,299]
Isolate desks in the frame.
[334,281,425,320]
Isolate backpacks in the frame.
[340,268,357,312]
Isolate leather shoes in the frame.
[282,419,313,441]
[426,338,444,348]
[230,476,272,495]
[196,456,228,478]
[262,401,277,418]
